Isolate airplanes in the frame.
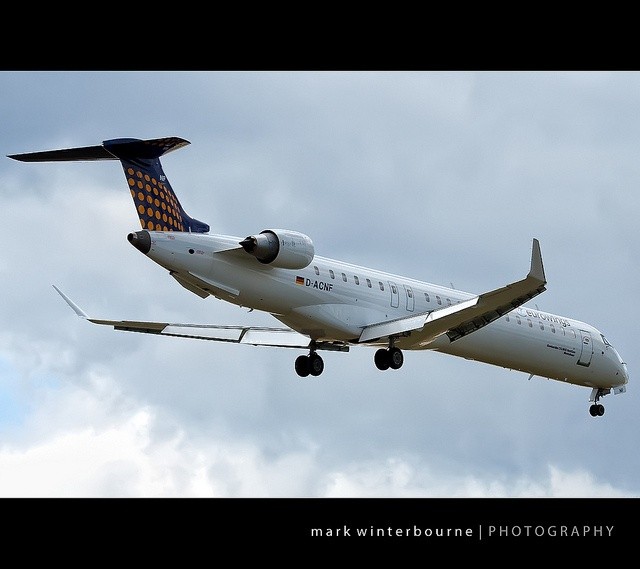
[3,136,629,417]
